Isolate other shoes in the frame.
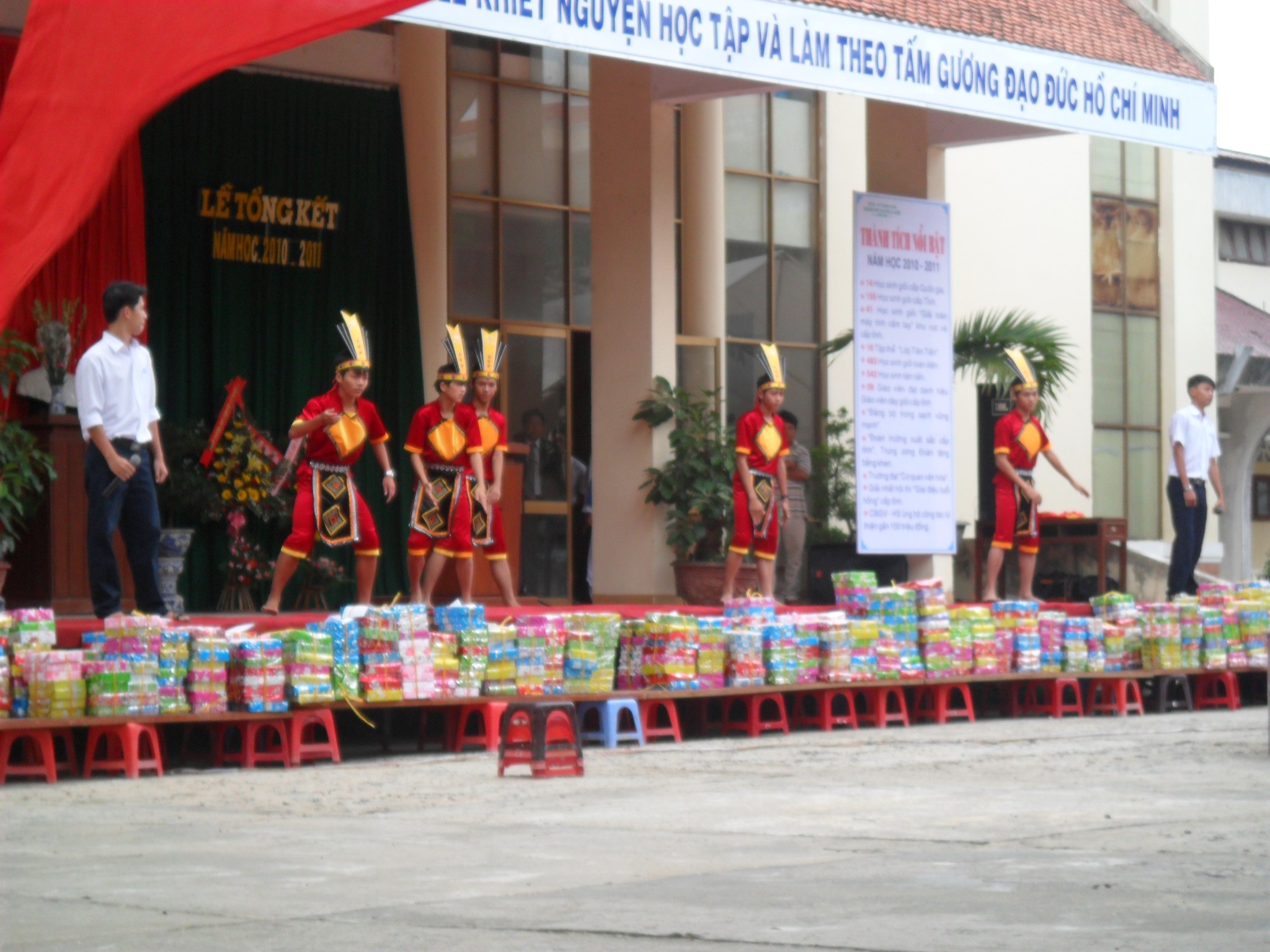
[1170,592,1188,600]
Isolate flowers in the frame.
[202,422,350,588]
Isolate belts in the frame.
[114,438,145,452]
[1189,477,1206,487]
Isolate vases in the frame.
[152,528,192,614]
[215,580,258,615]
[291,585,333,613]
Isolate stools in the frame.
[0,712,339,788]
[444,671,1243,780]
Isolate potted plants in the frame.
[49,386,66,415]
[632,376,762,602]
[0,327,60,598]
[801,405,907,604]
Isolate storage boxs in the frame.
[0,573,1270,718]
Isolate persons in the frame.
[72,280,169,623]
[511,411,593,605]
[1166,375,1225,602]
[984,375,1089,602]
[756,410,811,606]
[720,373,793,607]
[261,352,395,613]
[15,321,77,414]
[424,364,518,607]
[403,361,488,606]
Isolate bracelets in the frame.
[781,495,788,500]
[1183,485,1191,490]
[384,470,395,477]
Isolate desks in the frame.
[972,517,1127,604]
[17,414,136,615]
